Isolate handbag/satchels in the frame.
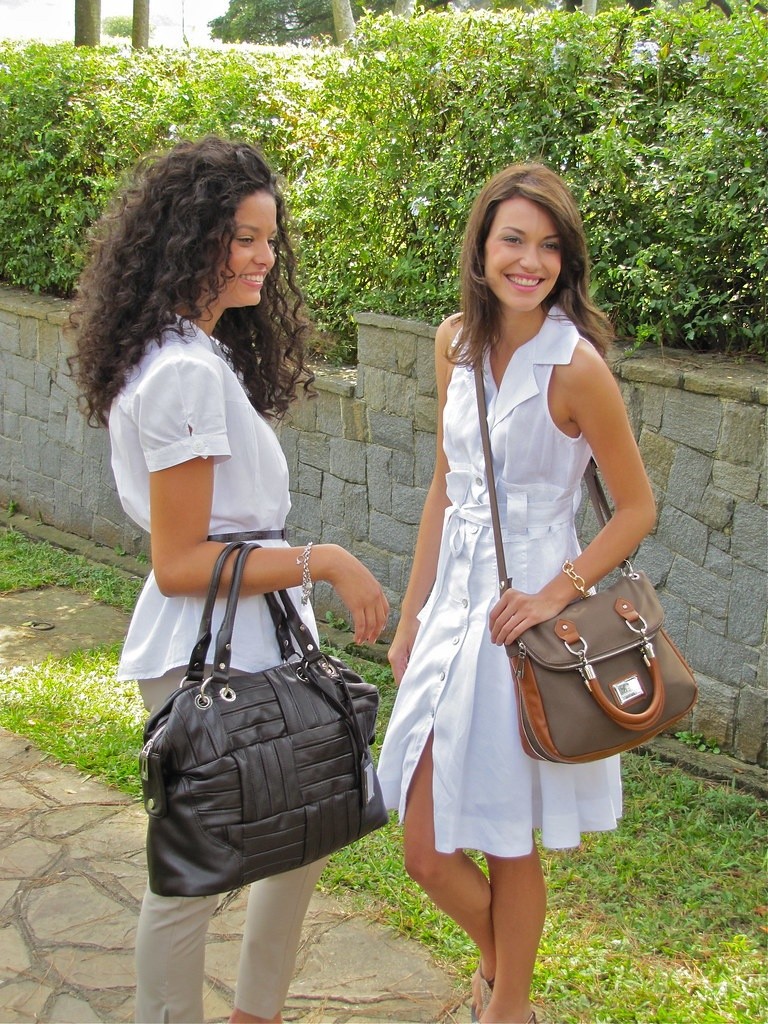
[138,540,389,896]
[506,568,698,762]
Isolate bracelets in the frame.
[296,542,315,606]
[563,557,591,601]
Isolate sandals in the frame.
[472,959,538,1024]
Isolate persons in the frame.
[374,161,658,1024]
[60,139,391,1024]
